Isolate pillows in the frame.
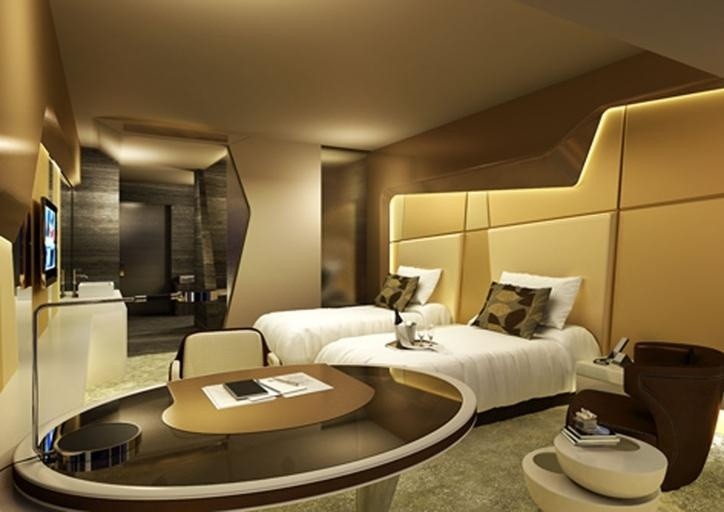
[395,262,445,305]
[472,281,553,340]
[496,269,586,330]
[373,270,421,313]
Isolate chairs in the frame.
[168,325,281,383]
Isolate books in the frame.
[200,371,335,410]
[560,422,621,446]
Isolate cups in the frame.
[403,324,414,345]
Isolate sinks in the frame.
[79,281,114,297]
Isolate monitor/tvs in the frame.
[39,196,58,290]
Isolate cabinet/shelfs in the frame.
[58,304,129,389]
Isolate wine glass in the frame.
[418,323,435,347]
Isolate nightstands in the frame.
[575,354,628,395]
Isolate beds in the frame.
[251,302,453,365]
[312,320,605,429]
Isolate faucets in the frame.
[72,269,88,293]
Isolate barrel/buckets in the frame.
[398,323,415,348]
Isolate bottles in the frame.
[393,304,406,342]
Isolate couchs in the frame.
[566,338,724,493]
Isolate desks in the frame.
[10,362,479,511]
[520,431,669,512]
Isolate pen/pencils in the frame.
[275,378,298,387]
